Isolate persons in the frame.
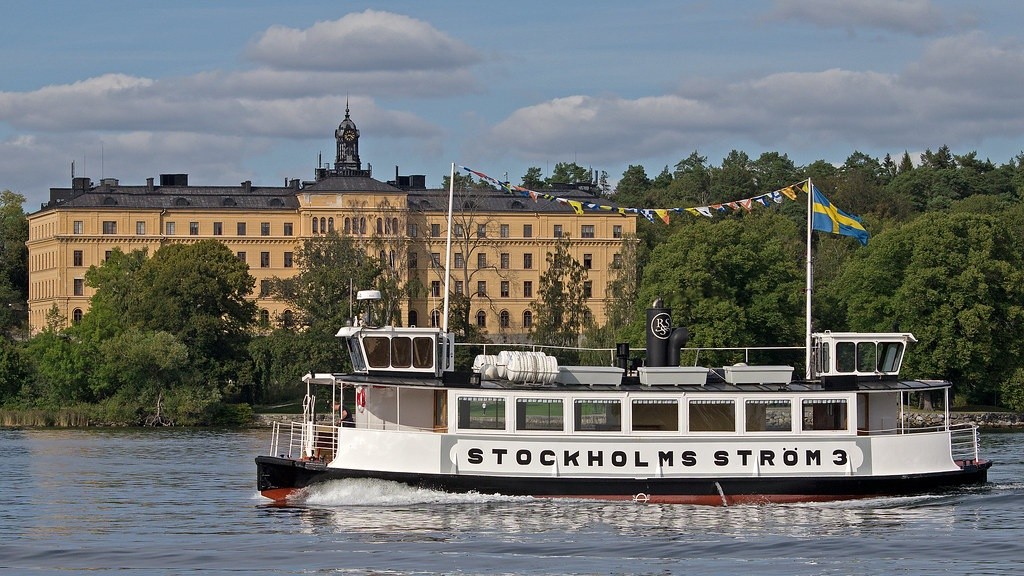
[482,402,486,415]
[330,401,353,428]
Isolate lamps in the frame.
[467,372,481,388]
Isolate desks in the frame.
[316,425,337,460]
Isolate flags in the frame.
[464,167,808,225]
[812,184,868,243]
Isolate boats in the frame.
[254,164,993,506]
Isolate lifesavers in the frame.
[356,385,365,413]
